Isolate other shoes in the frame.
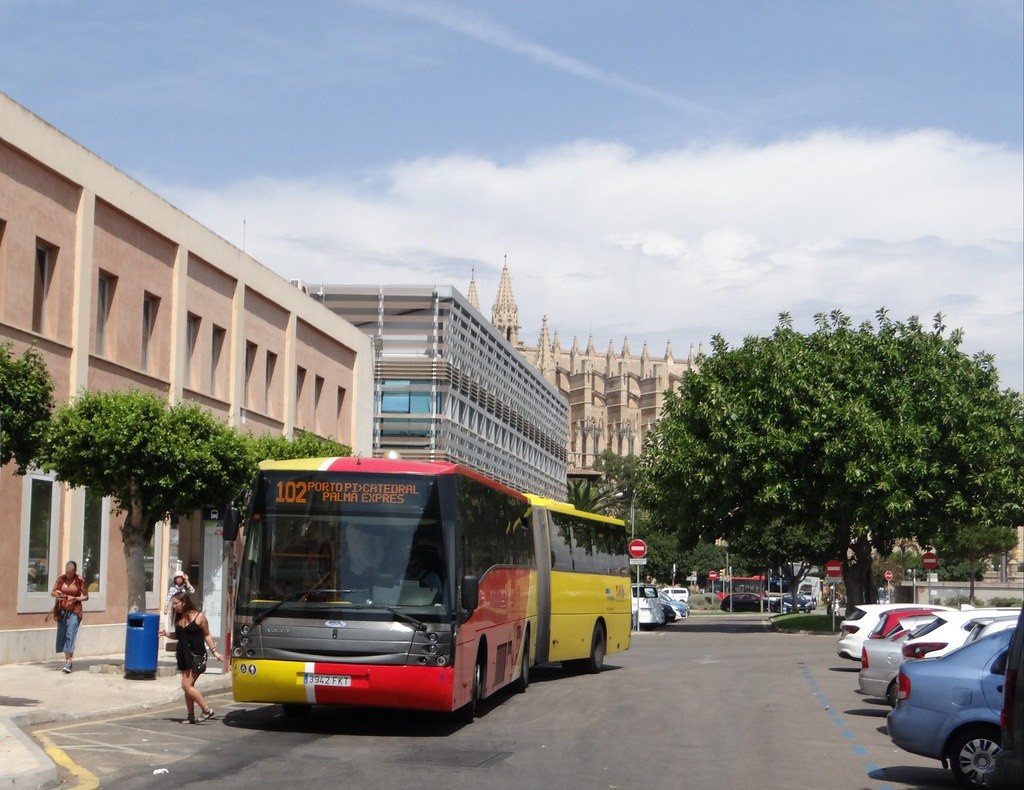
[63,663,72,673]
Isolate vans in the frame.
[662,588,689,603]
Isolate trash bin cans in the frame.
[124,611,161,681]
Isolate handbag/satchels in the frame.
[192,653,206,673]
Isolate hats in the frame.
[173,571,184,578]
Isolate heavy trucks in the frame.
[768,576,820,610]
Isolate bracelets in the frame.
[211,647,217,653]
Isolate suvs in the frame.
[901,608,1022,662]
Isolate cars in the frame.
[983,601,1024,790]
[658,590,690,622]
[720,593,774,613]
[775,593,812,614]
[962,615,1020,647]
[886,627,1017,790]
[835,603,960,661]
[632,583,664,630]
[857,609,947,709]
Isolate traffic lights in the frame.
[719,568,725,577]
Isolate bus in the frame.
[719,575,766,601]
[222,456,632,724]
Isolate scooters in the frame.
[827,598,840,617]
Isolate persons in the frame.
[164,570,195,630]
[345,523,385,581]
[158,592,224,723]
[51,561,89,673]
[397,551,443,599]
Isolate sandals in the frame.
[182,718,196,724]
[198,707,214,722]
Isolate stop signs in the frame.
[825,560,842,576]
[921,553,938,569]
[709,571,717,580]
[629,539,647,558]
[885,571,893,580]
